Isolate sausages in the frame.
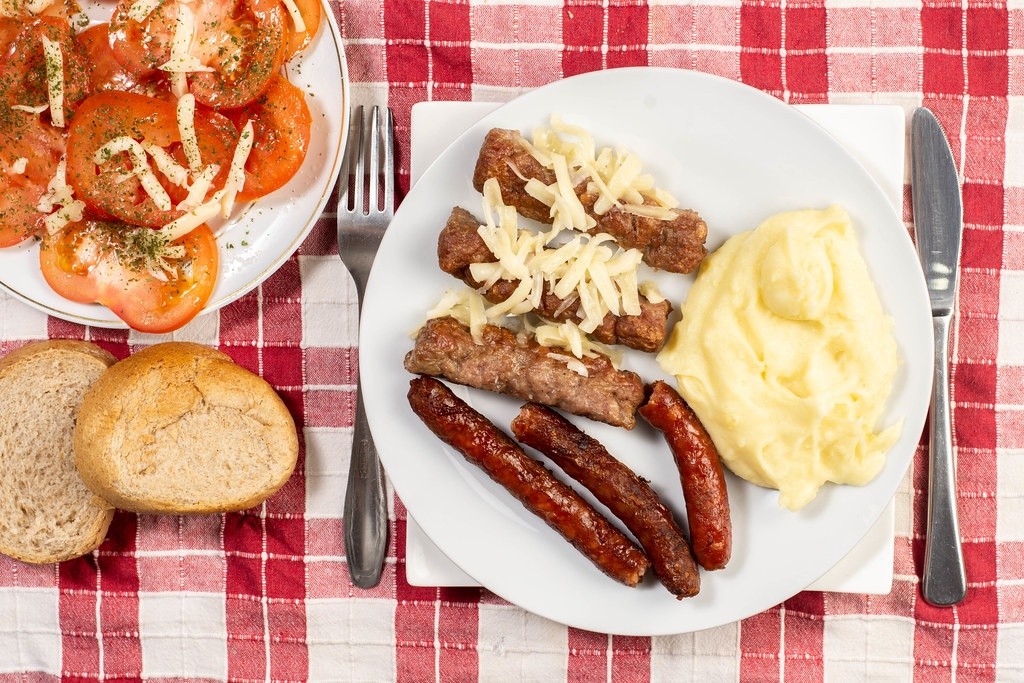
[408,376,732,600]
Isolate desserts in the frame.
[657,208,904,511]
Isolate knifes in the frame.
[910,108,968,609]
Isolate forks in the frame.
[335,105,395,590]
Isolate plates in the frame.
[0,1,351,330]
[358,64,935,637]
[406,98,904,600]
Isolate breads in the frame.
[74,342,299,514]
[0,339,120,563]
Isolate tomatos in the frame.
[0,0,320,333]
